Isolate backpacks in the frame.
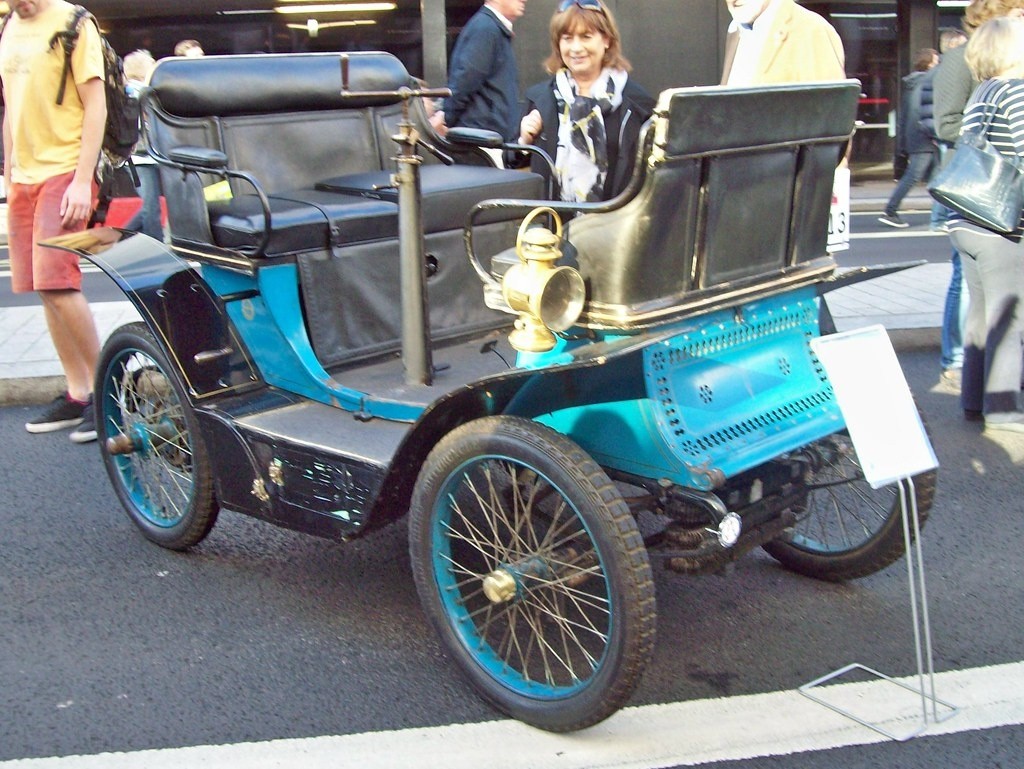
[0,4,146,188]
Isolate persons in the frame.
[878,27,970,233]
[505,0,657,201]
[173,40,206,56]
[718,0,852,169]
[0,1,108,443]
[428,0,525,144]
[931,0,1024,431]
[123,48,163,241]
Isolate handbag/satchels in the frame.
[925,84,1024,232]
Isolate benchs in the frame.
[461,78,867,359]
[136,52,562,371]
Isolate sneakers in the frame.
[877,212,909,227]
[25,391,89,433]
[68,392,97,442]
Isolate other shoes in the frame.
[930,224,949,232]
[963,409,982,421]
[939,369,962,395]
[984,419,1024,433]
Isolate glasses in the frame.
[557,0,604,14]
